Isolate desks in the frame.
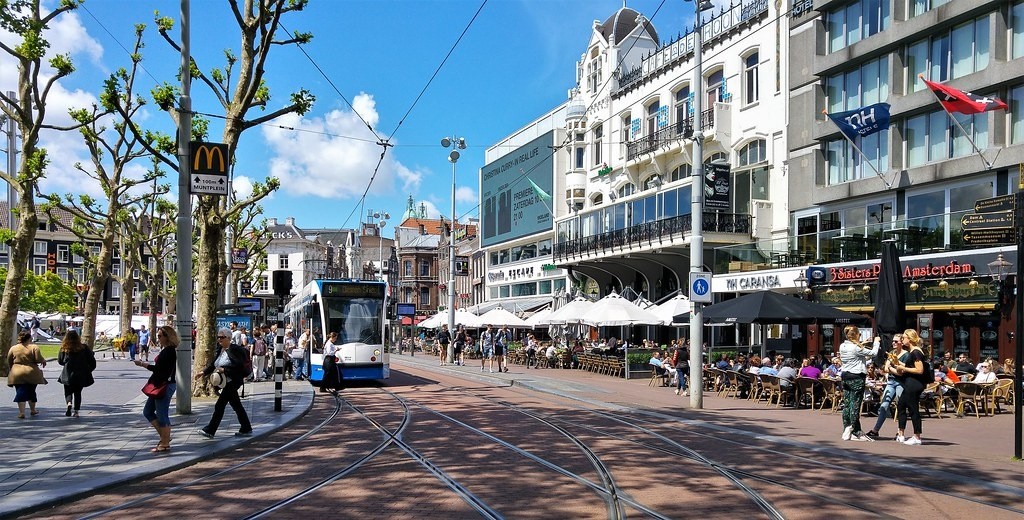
[831,235,867,262]
[883,228,922,253]
[774,253,805,268]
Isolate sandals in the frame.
[150,438,172,452]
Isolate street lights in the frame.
[373,210,390,282]
[441,135,478,360]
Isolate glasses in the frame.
[981,366,989,368]
[158,334,164,339]
[892,340,902,344]
[217,335,227,339]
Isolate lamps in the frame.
[804,284,811,294]
[608,191,617,201]
[910,276,919,290]
[969,273,979,289]
[987,249,1014,283]
[826,284,833,295]
[794,270,809,299]
[847,281,855,293]
[652,175,661,187]
[862,279,870,293]
[571,205,579,212]
[938,274,949,289]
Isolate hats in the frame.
[210,370,226,388]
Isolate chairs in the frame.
[507,344,1015,421]
[769,226,928,269]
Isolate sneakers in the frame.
[903,435,922,445]
[866,430,879,438]
[894,433,905,442]
[850,431,875,442]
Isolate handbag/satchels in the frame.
[292,347,304,359]
[142,382,166,397]
[495,343,503,355]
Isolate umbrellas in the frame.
[579,290,663,341]
[417,314,438,328]
[441,308,478,330]
[422,309,449,329]
[524,306,554,340]
[646,290,706,342]
[478,307,531,329]
[630,295,657,341]
[546,293,594,340]
[873,238,905,337]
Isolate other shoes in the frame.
[320,390,329,393]
[66,405,71,417]
[74,413,79,417]
[235,430,252,436]
[31,410,39,415]
[196,430,213,438]
[439,361,465,367]
[18,414,25,419]
[481,366,509,374]
[674,388,688,396]
[245,373,305,382]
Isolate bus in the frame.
[283,259,393,382]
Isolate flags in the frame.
[827,102,893,143]
[924,79,1009,115]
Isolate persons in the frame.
[6,330,47,419]
[56,330,97,417]
[21,316,54,343]
[396,325,1024,446]
[130,325,180,452]
[94,325,151,362]
[192,320,342,440]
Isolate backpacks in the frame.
[254,338,266,356]
[231,345,254,377]
[922,360,935,384]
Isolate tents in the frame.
[673,291,869,361]
